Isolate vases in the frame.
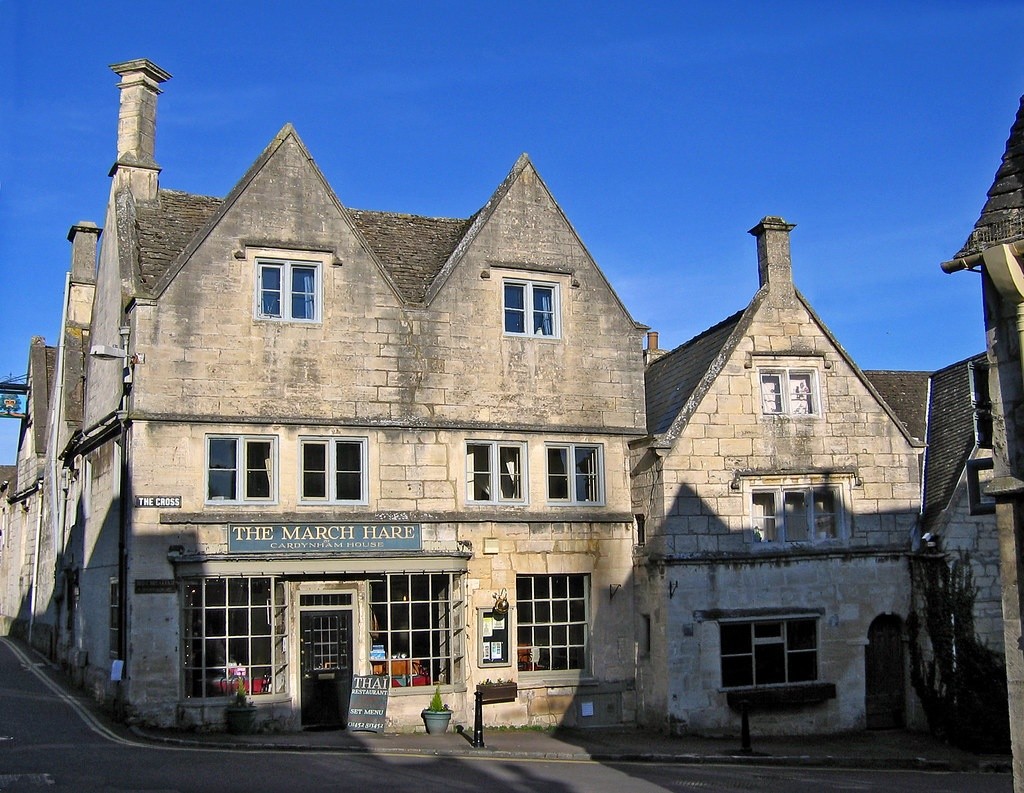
[476,682,518,705]
[422,710,454,735]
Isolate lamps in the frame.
[459,540,473,553]
[89,345,145,364]
[168,545,184,559]
[492,587,510,615]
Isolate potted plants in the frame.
[224,678,257,734]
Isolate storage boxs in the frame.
[386,660,406,675]
[411,676,430,686]
[196,675,225,697]
[221,676,244,695]
[373,645,384,651]
[371,651,385,660]
[243,676,263,693]
[392,679,406,687]
[408,661,420,674]
[374,665,383,675]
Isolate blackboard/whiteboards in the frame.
[346,673,390,730]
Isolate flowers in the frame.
[422,685,450,712]
[479,678,514,686]
